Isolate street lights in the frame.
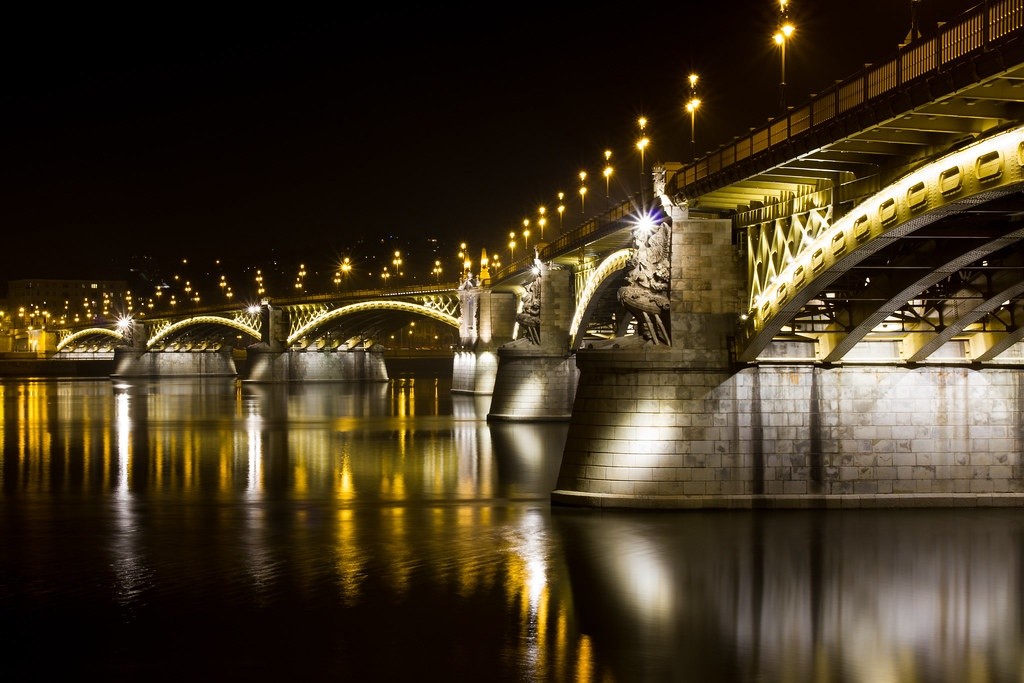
[558,193,564,237]
[433,260,442,281]
[299,264,307,297]
[459,243,466,285]
[185,282,200,308]
[603,151,613,210]
[539,207,545,240]
[523,219,529,257]
[772,0,795,117]
[393,251,402,296]
[255,270,265,301]
[342,258,351,293]
[381,267,390,283]
[295,278,302,297]
[509,232,515,264]
[219,276,233,304]
[579,172,587,223]
[636,118,649,188]
[492,255,500,274]
[334,273,341,288]
[685,75,700,164]
